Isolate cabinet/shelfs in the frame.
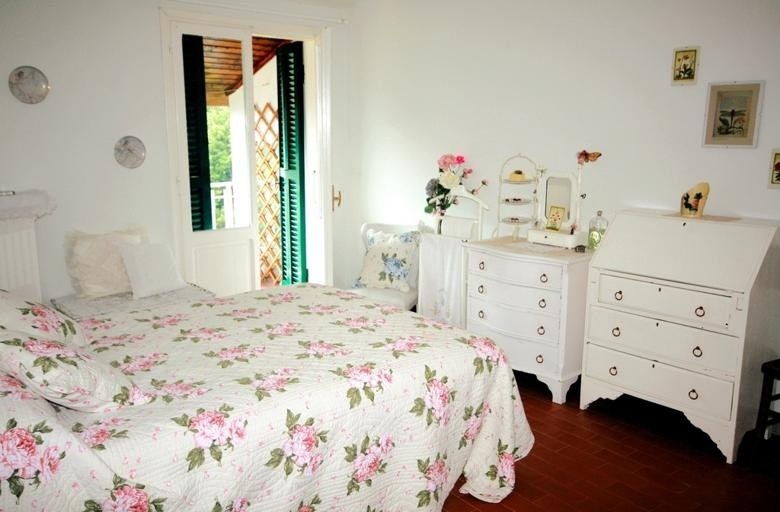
[467,234,594,405]
[578,205,780,465]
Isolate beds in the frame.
[0,282,537,511]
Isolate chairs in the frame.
[346,222,433,313]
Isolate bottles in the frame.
[587,210,610,250]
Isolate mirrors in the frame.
[539,171,578,228]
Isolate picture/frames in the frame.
[700,79,767,150]
[766,148,780,189]
[670,46,701,86]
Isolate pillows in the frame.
[62,226,149,301]
[0,289,97,348]
[0,327,157,411]
[351,219,427,293]
[119,238,189,300]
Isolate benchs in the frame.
[50,280,217,323]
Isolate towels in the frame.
[416,232,471,331]
[440,215,477,244]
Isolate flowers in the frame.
[424,152,493,234]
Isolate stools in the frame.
[749,358,779,465]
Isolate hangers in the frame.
[429,170,489,210]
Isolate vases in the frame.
[435,204,447,235]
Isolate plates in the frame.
[115,136,147,169]
[8,65,50,105]
